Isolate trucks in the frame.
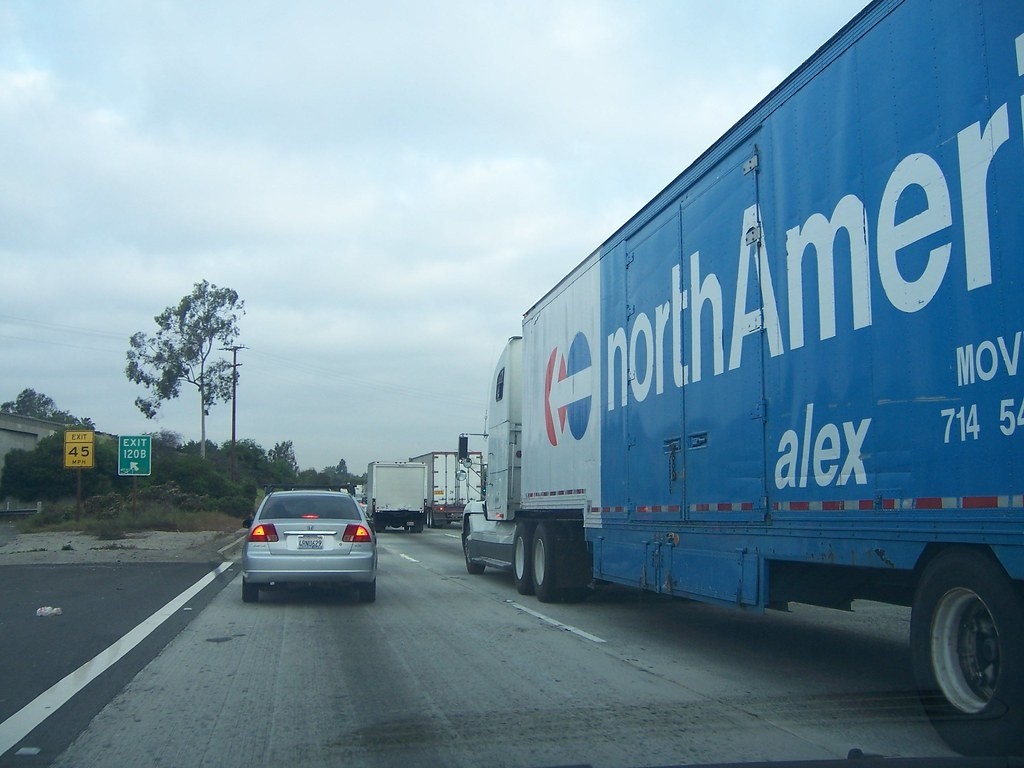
[361,461,429,534]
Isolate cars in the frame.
[242,483,382,603]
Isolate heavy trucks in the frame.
[457,0,1024,758]
[408,451,484,529]
